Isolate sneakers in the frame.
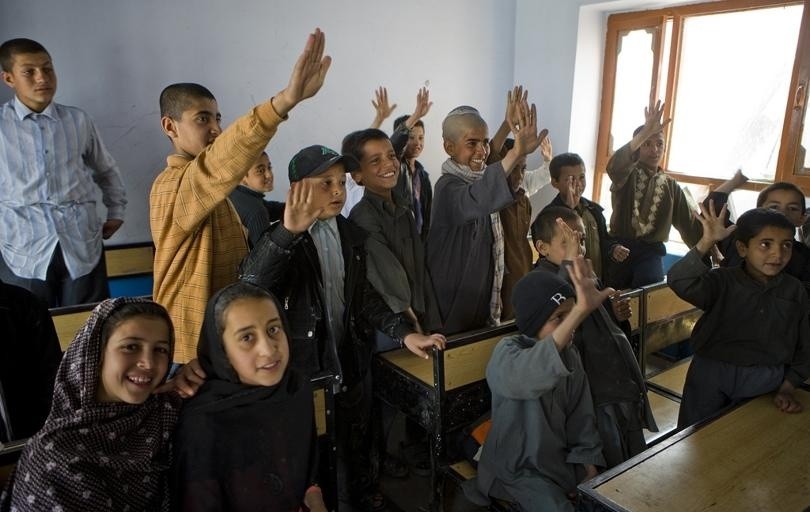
[380,453,410,480]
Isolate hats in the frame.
[510,270,577,337]
[288,145,357,185]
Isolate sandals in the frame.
[358,490,386,510]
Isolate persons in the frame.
[146,28,717,512]
[696,161,809,298]
[1,297,207,512]
[0,30,121,310]
[670,196,809,430]
[0,276,73,451]
